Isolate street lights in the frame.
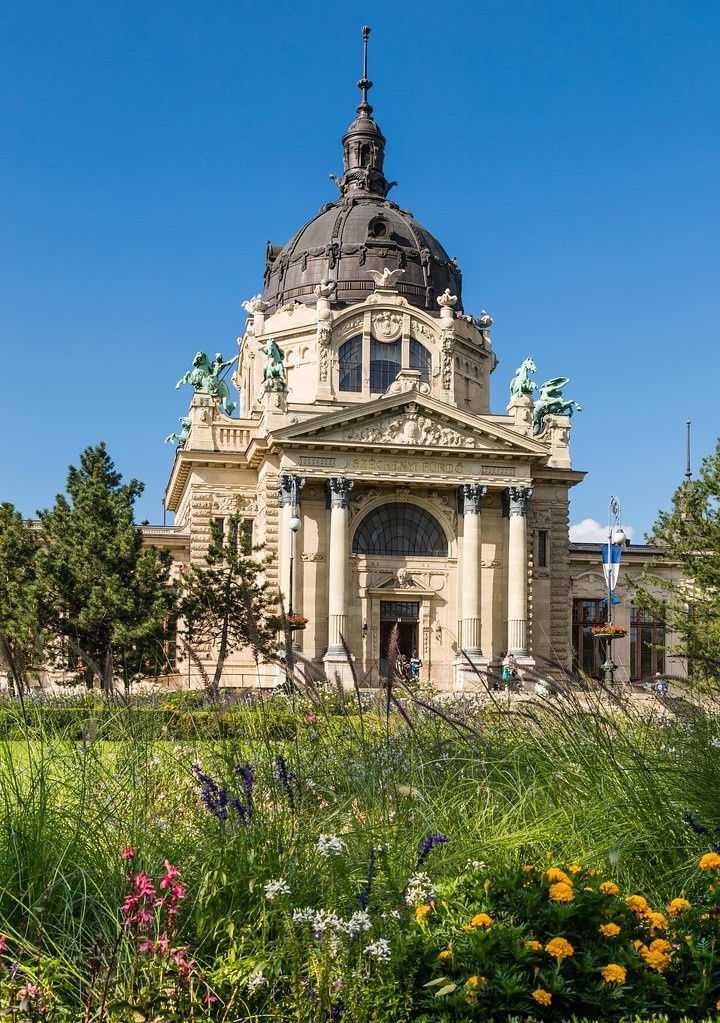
[599,496,627,697]
[281,513,303,694]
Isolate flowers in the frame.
[591,624,628,636]
[266,614,309,627]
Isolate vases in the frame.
[593,633,625,638]
[265,626,306,630]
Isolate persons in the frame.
[396,567,408,584]
[539,378,569,401]
[508,654,517,678]
[261,344,286,384]
[654,672,668,695]
[395,651,423,688]
[204,352,231,387]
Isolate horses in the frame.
[510,357,538,395]
[266,338,286,379]
[533,376,583,435]
[175,350,231,417]
[165,417,192,446]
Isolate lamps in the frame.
[363,618,368,635]
[436,620,442,638]
